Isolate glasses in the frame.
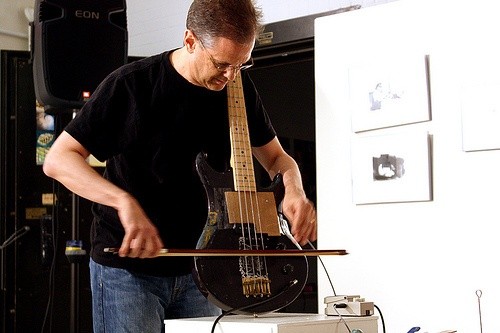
[195,35,254,70]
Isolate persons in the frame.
[42,1,318,333]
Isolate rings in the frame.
[311,218,315,224]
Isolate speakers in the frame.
[31,0,128,115]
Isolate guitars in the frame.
[189,66,310,319]
